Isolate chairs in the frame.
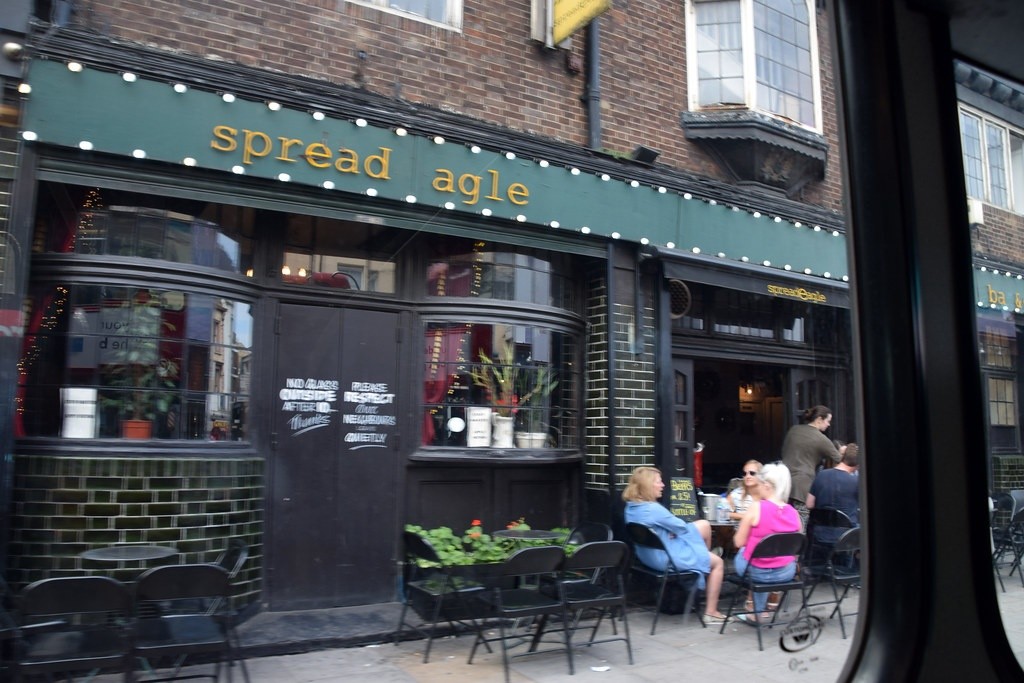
[991,488,1024,590]
[11,575,133,683]
[465,547,577,682]
[148,541,253,682]
[529,543,636,676]
[805,505,862,606]
[134,563,236,682]
[393,531,495,664]
[720,533,816,651]
[622,522,707,635]
[798,526,864,641]
[524,523,615,650]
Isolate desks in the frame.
[493,528,567,660]
[76,542,180,683]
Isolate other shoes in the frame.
[744,600,770,618]
[711,547,724,558]
[703,613,735,626]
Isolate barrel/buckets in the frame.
[697,492,721,521]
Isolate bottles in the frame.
[716,493,730,523]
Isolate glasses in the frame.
[741,471,758,476]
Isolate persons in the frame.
[733,462,804,627]
[805,442,861,542]
[728,460,768,520]
[817,439,859,477]
[780,405,849,531]
[624,466,736,624]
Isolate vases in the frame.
[467,404,494,448]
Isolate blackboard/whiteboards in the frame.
[668,476,701,522]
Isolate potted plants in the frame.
[96,290,178,444]
[512,360,558,449]
[464,336,539,450]
